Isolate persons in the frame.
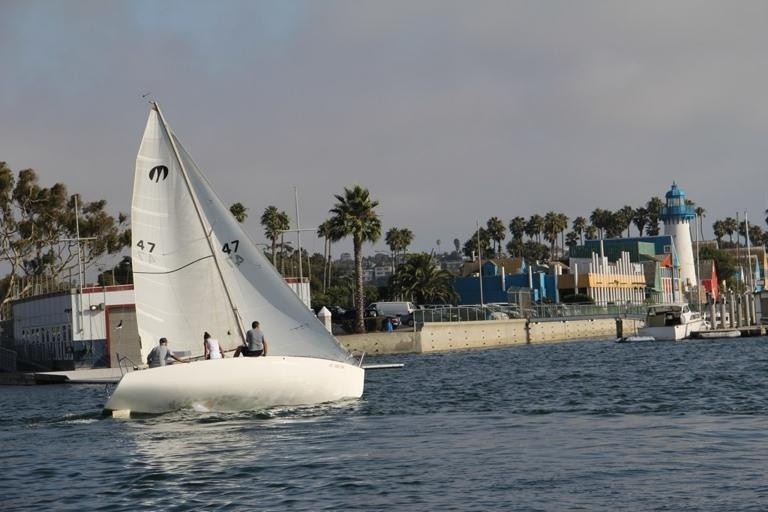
[233,320,267,357]
[204,331,225,359]
[147,337,184,367]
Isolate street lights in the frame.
[374,248,409,276]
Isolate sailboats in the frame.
[92,87,372,416]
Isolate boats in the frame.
[705,312,740,330]
[697,331,743,339]
[615,336,656,344]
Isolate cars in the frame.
[318,301,571,331]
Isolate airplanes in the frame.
[636,301,710,344]
